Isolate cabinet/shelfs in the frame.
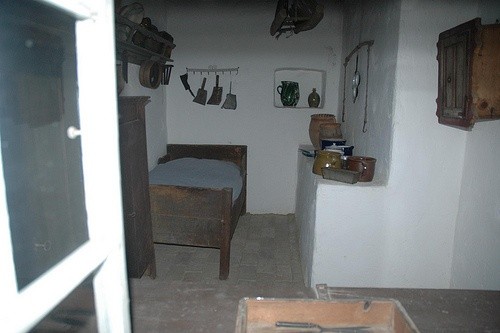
[118,96,156,279]
[436,17,500,131]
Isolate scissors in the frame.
[276,322,371,333]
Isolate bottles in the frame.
[308,88,320,108]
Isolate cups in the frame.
[277,81,300,106]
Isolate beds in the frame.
[150,144,247,280]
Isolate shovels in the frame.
[207,75,223,105]
[221,81,237,110]
[193,78,207,106]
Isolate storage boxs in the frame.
[235,296,420,333]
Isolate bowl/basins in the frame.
[347,156,377,182]
[312,151,342,176]
